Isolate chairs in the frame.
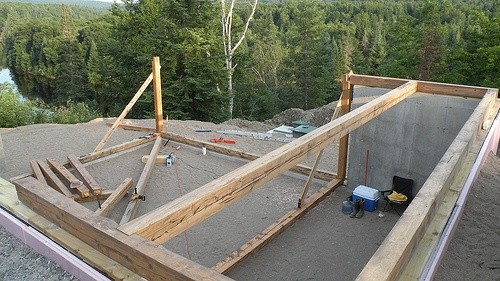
[378,176,414,217]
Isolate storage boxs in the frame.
[352,184,381,212]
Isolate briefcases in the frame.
[353,184,380,211]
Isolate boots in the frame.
[349,198,366,217]
